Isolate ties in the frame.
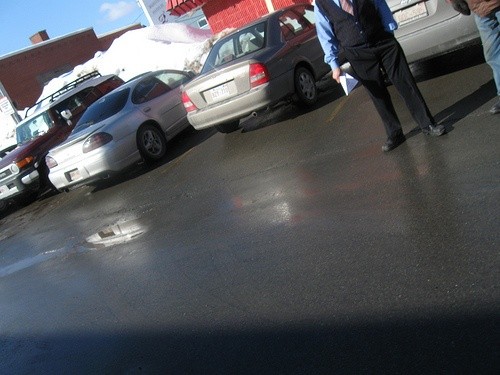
[339,0,354,16]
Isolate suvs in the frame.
[0,70,127,205]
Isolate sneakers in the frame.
[381,133,405,152]
[488,97,500,113]
[424,124,445,136]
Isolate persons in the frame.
[314,0,446,152]
[449,0,500,114]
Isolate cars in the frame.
[45,69,200,195]
[181,1,347,133]
[386,0,491,61]
